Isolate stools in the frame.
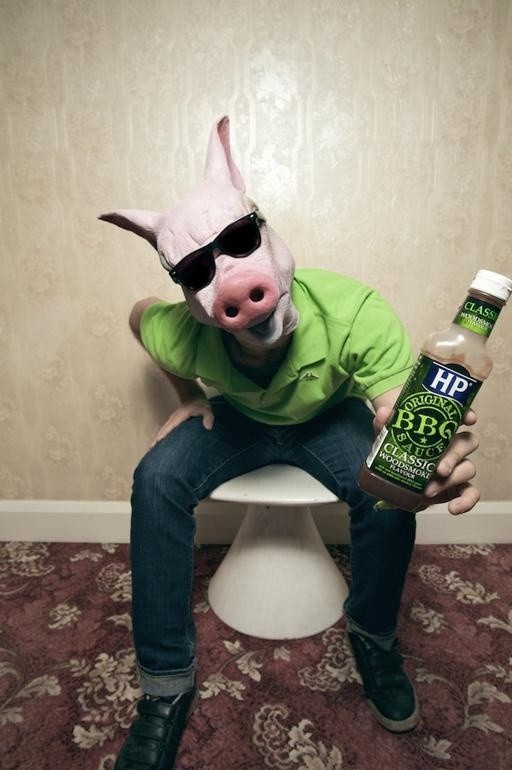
[205,467,351,645]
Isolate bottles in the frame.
[354,269,512,513]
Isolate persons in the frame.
[93,113,485,770]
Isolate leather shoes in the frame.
[114,671,200,770]
[349,628,420,733]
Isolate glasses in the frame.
[160,212,264,292]
